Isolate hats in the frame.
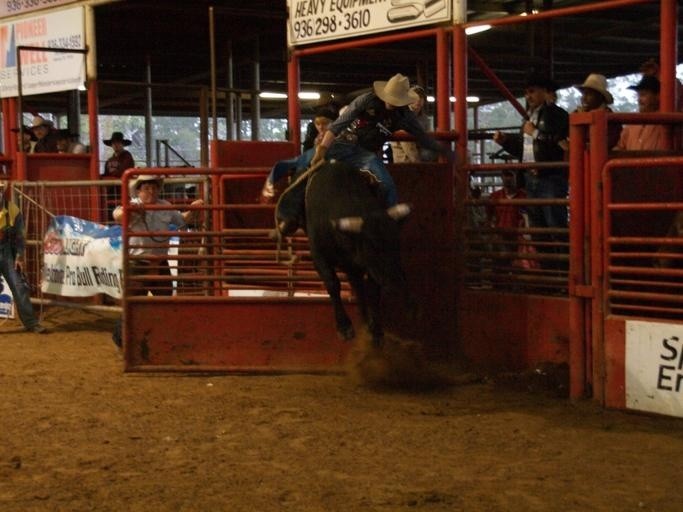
[103,132,131,146]
[372,73,420,106]
[625,74,660,93]
[574,74,614,104]
[26,116,54,131]
[129,173,165,197]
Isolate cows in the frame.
[303,147,421,351]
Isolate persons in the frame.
[264,74,682,284]
[102,132,135,228]
[11,117,87,154]
[113,173,205,350]
[1,154,49,333]
